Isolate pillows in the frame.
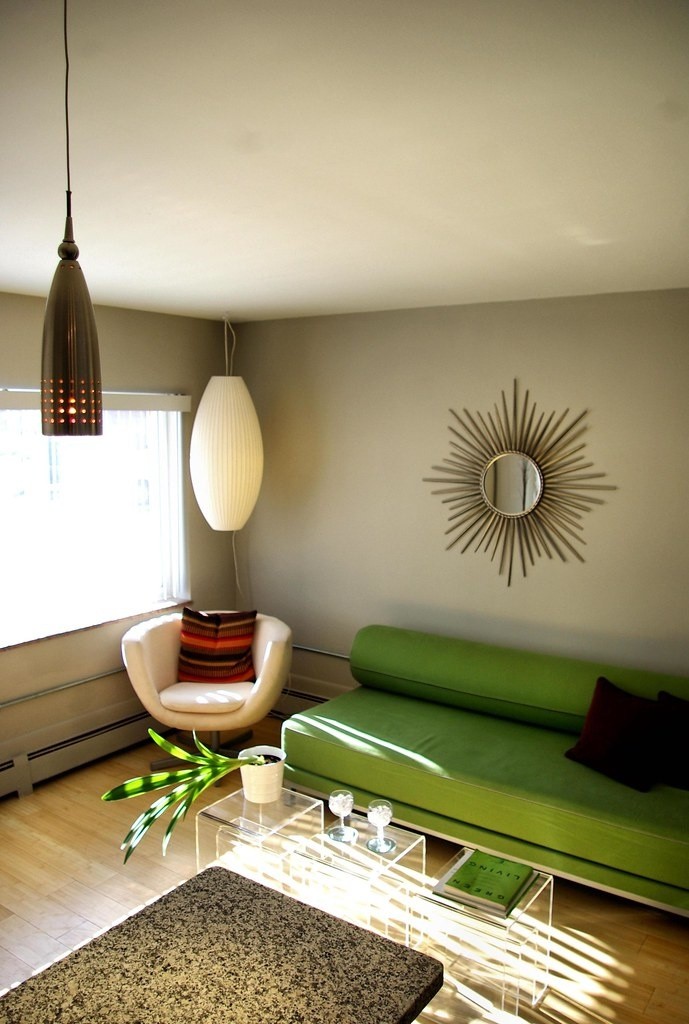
[563,675,672,792]
[654,687,689,791]
[175,605,257,684]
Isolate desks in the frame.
[0,866,443,1024]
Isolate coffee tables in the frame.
[197,785,555,1017]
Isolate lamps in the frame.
[189,312,263,598]
[37,0,103,438]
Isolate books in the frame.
[432,849,541,920]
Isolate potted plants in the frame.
[100,724,287,863]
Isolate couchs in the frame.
[280,623,689,922]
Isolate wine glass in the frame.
[366,800,392,853]
[329,790,354,840]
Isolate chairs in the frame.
[121,611,293,788]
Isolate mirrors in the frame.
[479,451,544,520]
